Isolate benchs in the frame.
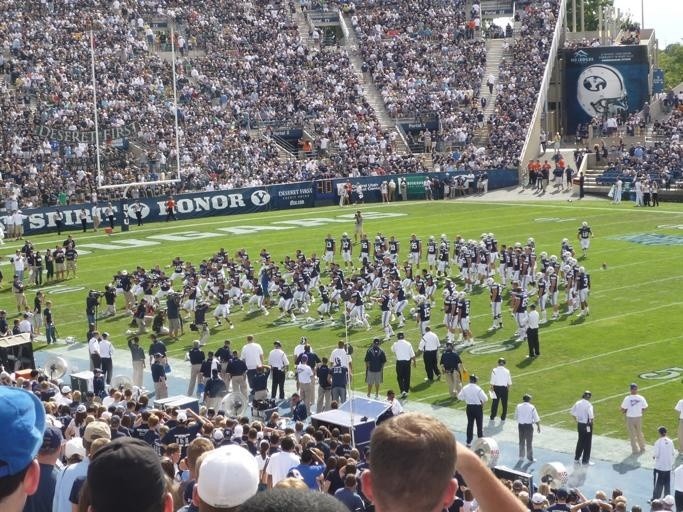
[247,402,278,418]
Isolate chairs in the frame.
[595,166,674,184]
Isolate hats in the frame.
[76,405,87,414]
[257,432,264,440]
[629,383,638,390]
[197,444,260,509]
[83,421,112,444]
[0,386,48,478]
[658,426,666,432]
[528,304,536,308]
[556,487,568,499]
[662,495,675,505]
[41,425,63,452]
[65,437,87,460]
[335,357,342,365]
[177,412,188,423]
[213,430,224,440]
[274,341,281,347]
[62,386,72,394]
[46,414,62,428]
[154,352,164,360]
[87,436,166,512]
[234,424,243,437]
[300,336,307,344]
[532,492,546,504]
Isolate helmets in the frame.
[452,291,458,299]
[563,252,572,261]
[582,391,592,398]
[480,233,487,239]
[527,237,535,246]
[459,239,466,246]
[546,266,555,275]
[566,257,573,264]
[535,272,543,281]
[563,265,571,274]
[417,296,426,304]
[550,255,557,264]
[582,222,588,228]
[458,291,466,299]
[540,252,548,261]
[488,233,494,238]
[467,239,472,247]
[498,358,506,365]
[470,241,478,249]
[469,374,477,382]
[569,259,577,267]
[342,232,348,239]
[579,267,585,274]
[122,270,128,276]
[523,394,532,402]
[515,243,521,248]
[561,239,569,247]
[429,236,435,240]
[441,234,447,239]
[397,332,404,339]
[442,290,450,299]
[486,277,495,286]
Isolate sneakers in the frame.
[582,461,594,467]
[515,338,524,342]
[400,391,406,399]
[264,311,269,316]
[248,309,255,313]
[488,325,497,332]
[528,458,536,462]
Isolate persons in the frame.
[1,203,683,512]
[3,1,683,209]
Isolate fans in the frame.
[110,375,130,395]
[44,356,67,383]
[542,461,569,486]
[469,437,500,469]
[219,392,246,418]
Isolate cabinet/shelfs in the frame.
[70,371,104,394]
[355,441,369,456]
